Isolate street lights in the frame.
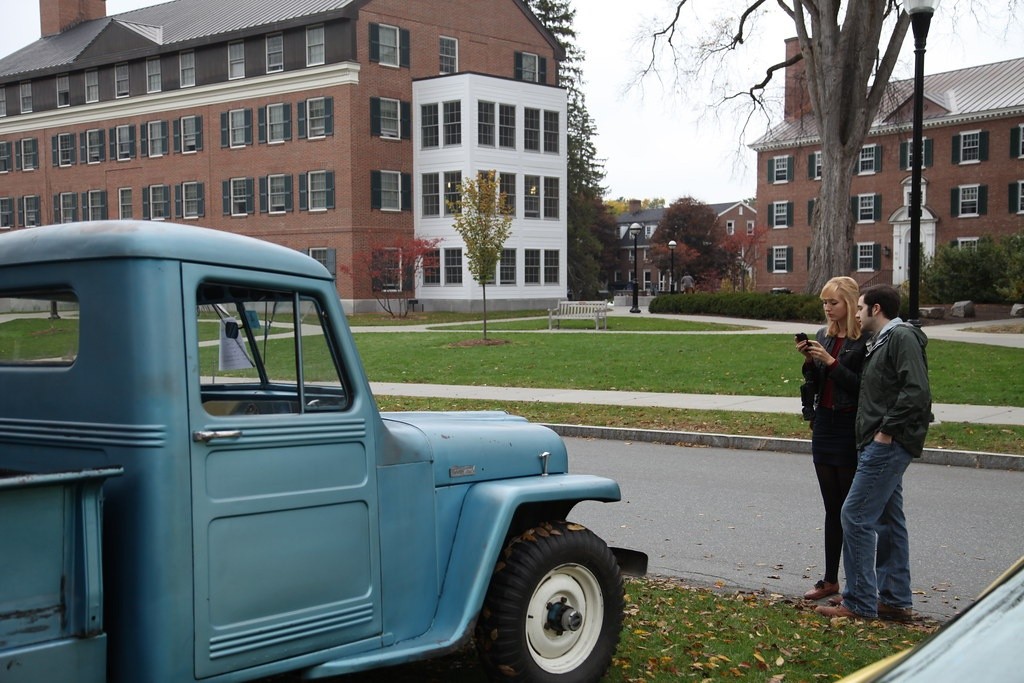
[668,240,677,294]
[630,222,643,313]
[903,1,940,327]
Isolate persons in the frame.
[814,284,932,623]
[794,277,872,600]
[681,272,694,294]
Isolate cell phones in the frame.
[796,333,812,352]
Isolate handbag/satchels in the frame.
[800,380,820,420]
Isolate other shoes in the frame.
[877,599,912,623]
[816,603,873,622]
[804,580,839,599]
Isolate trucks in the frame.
[0,220,648,683]
